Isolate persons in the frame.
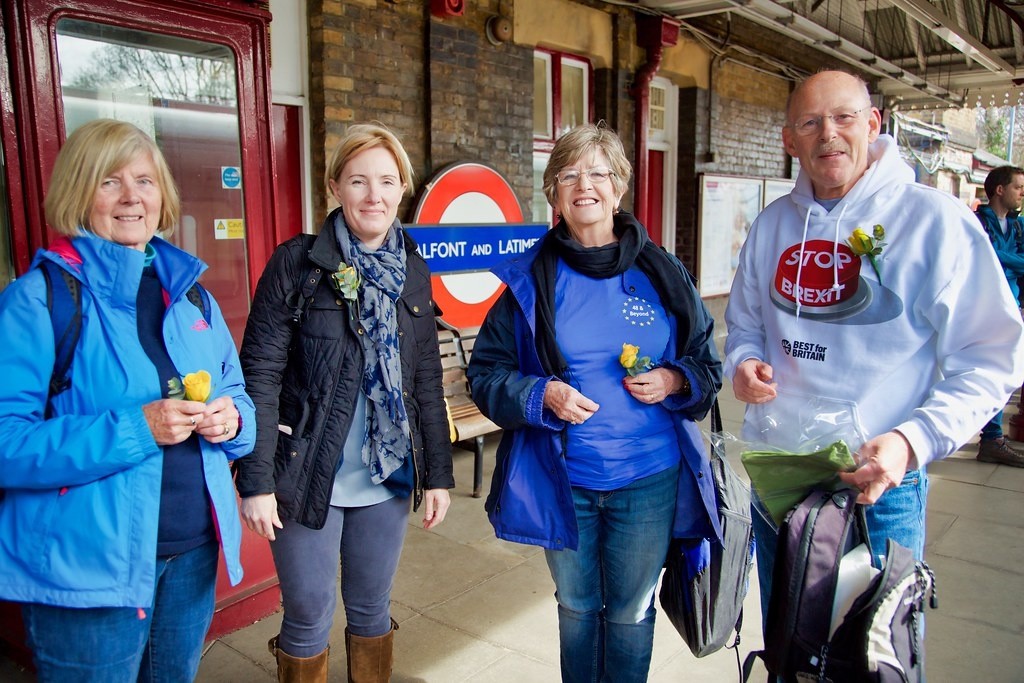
[235,125,455,683]
[0,120,256,683]
[465,124,724,683]
[973,166,1024,467]
[724,70,1024,682]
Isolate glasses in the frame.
[555,166,618,186]
[792,105,869,135]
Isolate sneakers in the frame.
[977,433,1024,467]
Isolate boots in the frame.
[344,616,400,683]
[269,633,330,683]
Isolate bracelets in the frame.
[679,372,691,393]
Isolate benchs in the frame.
[434,316,503,498]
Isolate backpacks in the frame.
[770,488,938,682]
[657,356,756,659]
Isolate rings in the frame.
[191,416,195,425]
[651,394,655,400]
[223,424,229,435]
[571,416,577,425]
